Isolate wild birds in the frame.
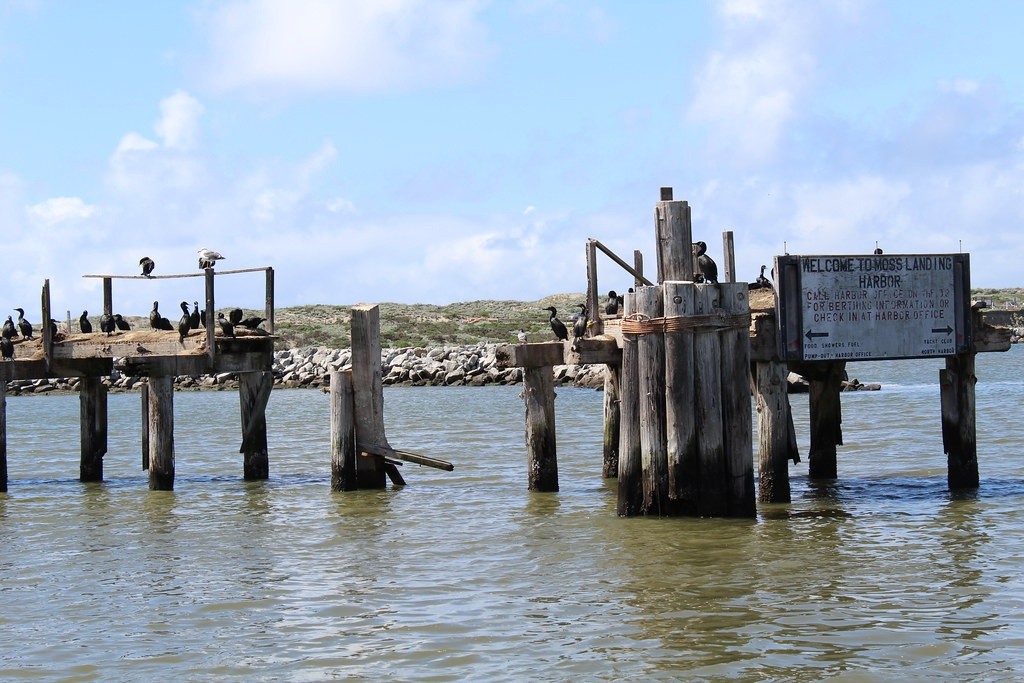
[692,241,720,288]
[542,288,636,341]
[756,265,772,288]
[0,247,267,361]
